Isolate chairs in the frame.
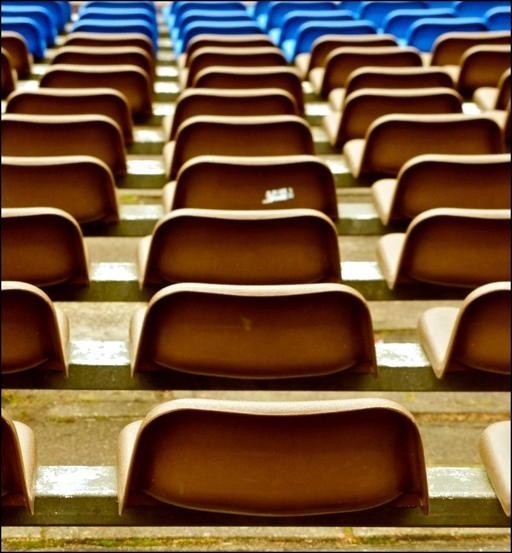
[109,398,431,526]
[1,1,512,177]
[137,210,345,292]
[128,283,380,389]
[377,206,511,292]
[2,204,93,295]
[1,412,37,521]
[418,280,511,392]
[480,417,512,522]
[371,153,512,227]
[158,154,341,224]
[0,155,123,225]
[1,279,72,386]
